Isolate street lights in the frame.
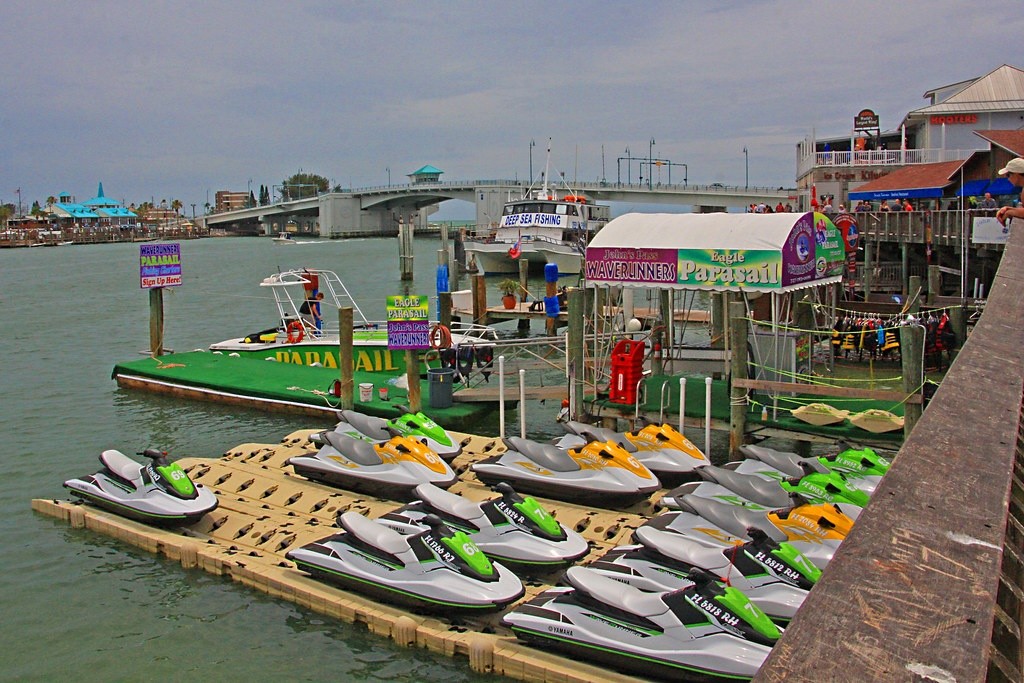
[297,165,303,201]
[741,146,749,190]
[625,147,631,187]
[648,135,655,191]
[248,178,253,211]
[386,166,391,190]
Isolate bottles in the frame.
[762,406,767,420]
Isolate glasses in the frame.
[1006,173,1013,178]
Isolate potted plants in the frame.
[497,278,520,309]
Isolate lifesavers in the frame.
[287,321,303,343]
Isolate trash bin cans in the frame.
[426,368,455,409]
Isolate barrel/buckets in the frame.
[359,382,374,403]
[379,389,388,400]
[427,368,455,408]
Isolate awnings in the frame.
[955,178,1021,196]
[847,159,965,200]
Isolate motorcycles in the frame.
[501,440,895,683]
[284,509,527,617]
[286,428,462,499]
[306,402,464,464]
[405,480,592,574]
[61,448,219,527]
[469,430,663,509]
[560,414,712,484]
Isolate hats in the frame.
[998,158,1024,175]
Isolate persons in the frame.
[300,293,324,339]
[819,204,827,212]
[879,199,891,211]
[855,201,871,212]
[903,200,913,211]
[838,205,846,212]
[994,158,1024,226]
[890,199,903,211]
[748,202,792,213]
[982,193,996,209]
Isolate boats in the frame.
[272,231,297,246]
[462,133,617,276]
[209,268,498,384]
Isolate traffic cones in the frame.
[528,138,538,186]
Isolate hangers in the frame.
[970,305,984,320]
[843,309,948,326]
